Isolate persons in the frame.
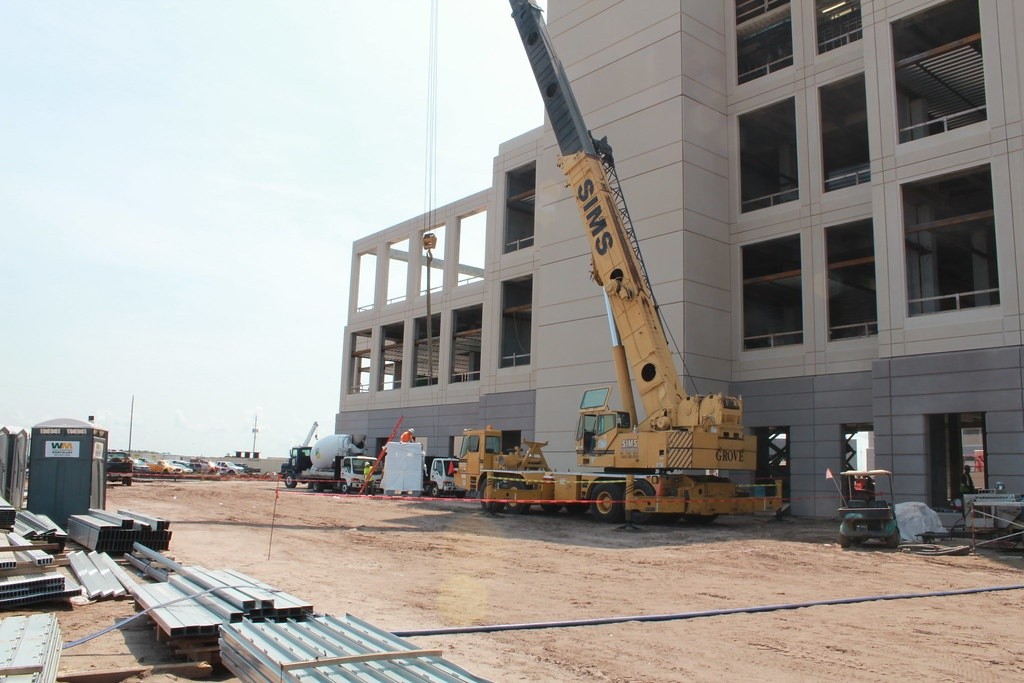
[400,428,415,444]
[959,465,975,494]
[363,462,375,495]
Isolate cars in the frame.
[132,456,263,478]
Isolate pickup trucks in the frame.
[105,450,134,487]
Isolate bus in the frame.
[823,468,901,549]
[276,415,469,498]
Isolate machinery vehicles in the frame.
[446,0,784,532]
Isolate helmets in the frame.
[365,462,369,466]
[409,428,414,434]
